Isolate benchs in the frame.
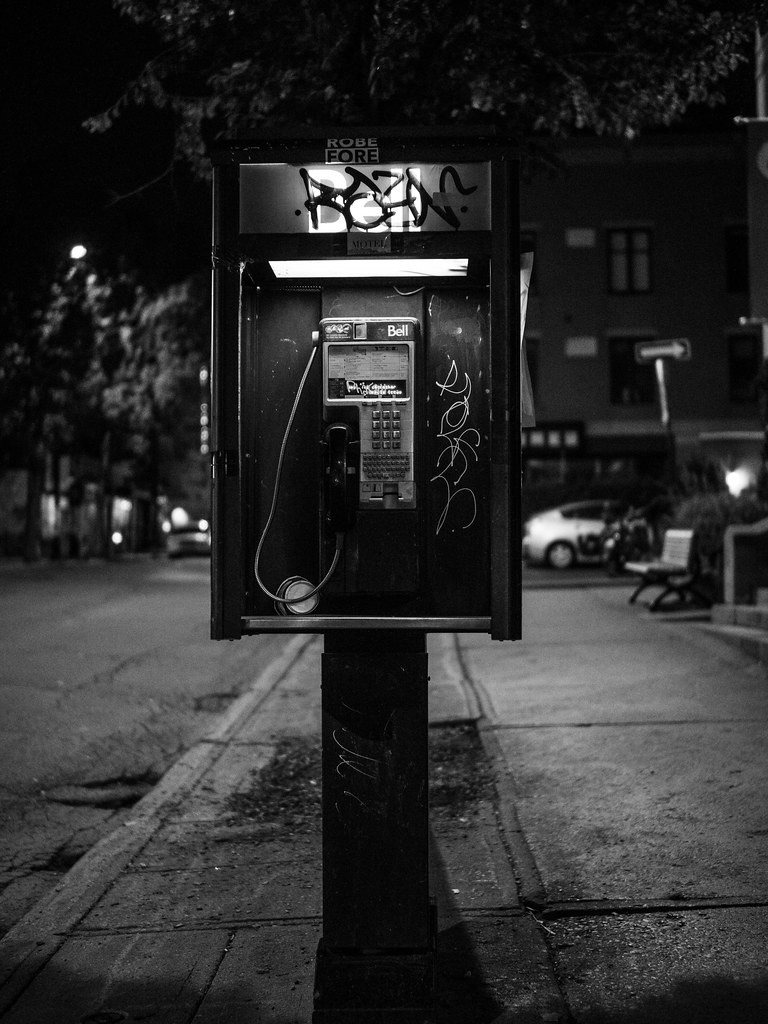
[624,526,709,612]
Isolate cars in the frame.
[524,499,654,570]
[166,524,210,556]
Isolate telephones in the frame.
[310,316,426,531]
